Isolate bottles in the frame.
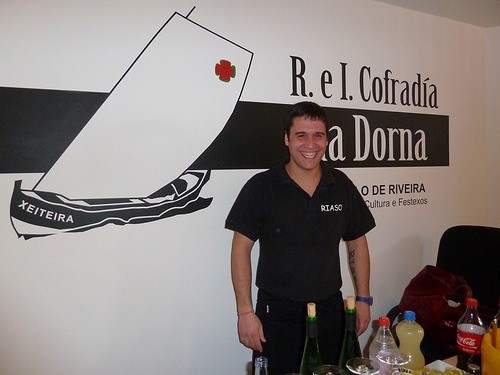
[395,311,425,375]
[456,299,486,375]
[338,296,363,368]
[299,303,323,375]
[369,316,400,375]
[254,356,268,375]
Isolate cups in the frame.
[480,326,500,375]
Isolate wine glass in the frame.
[312,364,347,375]
[345,357,380,375]
[377,350,412,375]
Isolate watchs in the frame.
[356,295,373,306]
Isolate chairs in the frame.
[386,226,500,366]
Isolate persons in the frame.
[224,102,376,375]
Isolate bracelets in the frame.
[237,309,254,316]
